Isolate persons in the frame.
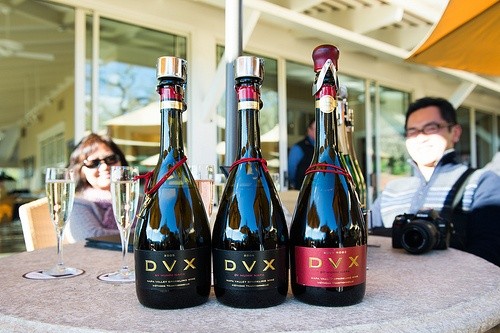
[367,97,500,267]
[63,132,144,242]
[287,117,316,190]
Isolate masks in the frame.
[405,134,454,165]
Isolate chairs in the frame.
[18,197,70,251]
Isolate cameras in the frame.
[392,209,450,254]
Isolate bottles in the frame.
[212,55,289,309]
[288,43,369,307]
[132,56,213,310]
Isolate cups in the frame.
[214,174,228,207]
[268,173,280,197]
[187,164,214,224]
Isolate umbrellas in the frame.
[403,0,500,78]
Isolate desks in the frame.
[0,235,500,333]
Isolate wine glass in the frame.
[43,166,77,277]
[109,165,140,280]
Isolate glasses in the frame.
[81,154,122,168]
[406,122,454,137]
